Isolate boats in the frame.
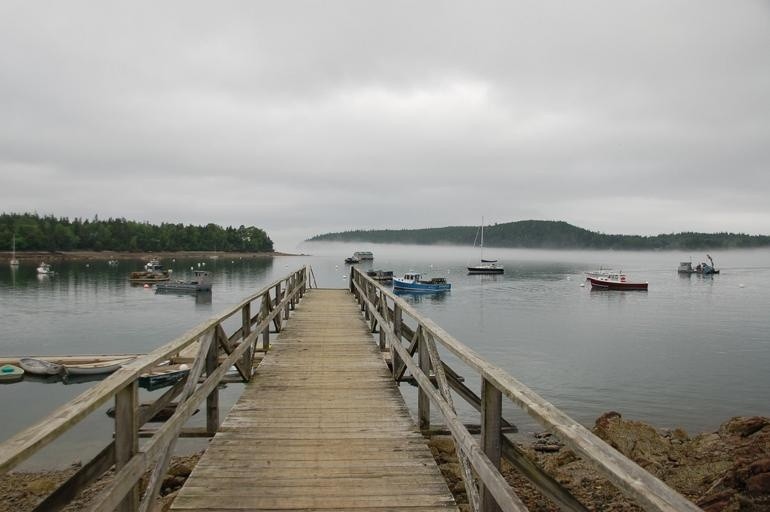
[344,251,374,264]
[391,272,452,293]
[128,257,173,285]
[365,269,394,284]
[36,260,56,274]
[108,258,120,266]
[155,270,213,292]
[677,254,720,274]
[585,266,649,290]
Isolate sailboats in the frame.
[10,232,20,265]
[466,215,506,274]
